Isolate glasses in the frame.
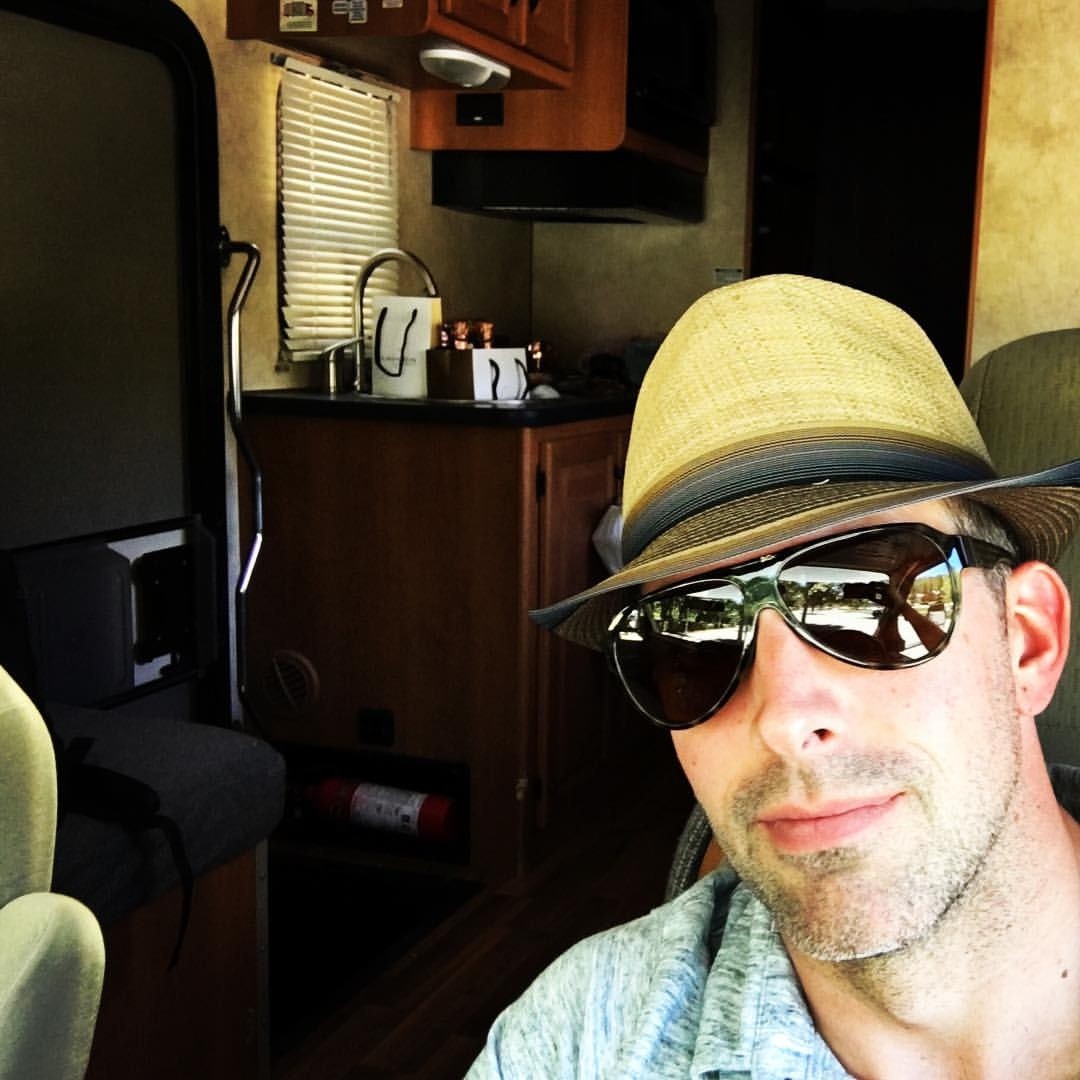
[608,521,1022,731]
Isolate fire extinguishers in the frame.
[288,772,457,843]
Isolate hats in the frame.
[524,269,1079,660]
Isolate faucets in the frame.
[352,248,439,394]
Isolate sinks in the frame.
[355,374,576,407]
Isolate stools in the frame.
[35,703,289,1080]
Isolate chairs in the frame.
[0,666,111,1077]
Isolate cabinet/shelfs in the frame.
[242,379,687,901]
[225,0,580,92]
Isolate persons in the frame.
[469,278,1080,1077]
[862,580,946,653]
